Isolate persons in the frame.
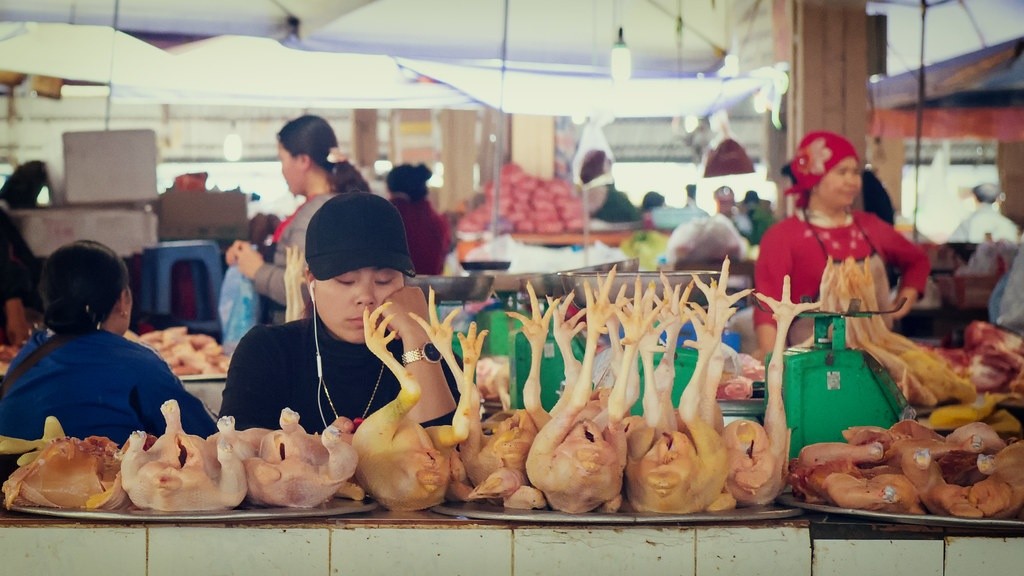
[0,240,219,448]
[219,194,463,428]
[580,150,797,236]
[948,183,1019,242]
[0,214,31,342]
[861,170,896,288]
[226,114,370,326]
[754,131,931,360]
[387,163,452,276]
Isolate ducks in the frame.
[122,397,251,512]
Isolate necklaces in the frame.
[321,361,385,421]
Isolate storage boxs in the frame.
[157,187,247,244]
[10,206,158,258]
[63,130,160,206]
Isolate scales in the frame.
[558,272,721,418]
[751,296,920,460]
[514,271,595,412]
[405,274,516,382]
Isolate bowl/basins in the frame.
[407,274,495,305]
[517,256,640,302]
[557,270,722,310]
[459,261,512,270]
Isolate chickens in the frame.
[348,286,493,513]
[2,415,130,511]
[246,405,364,510]
[793,426,930,516]
[888,418,1024,520]
[412,282,562,505]
[520,257,823,517]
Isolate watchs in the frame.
[402,342,442,367]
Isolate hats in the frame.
[305,189,416,281]
[783,131,861,208]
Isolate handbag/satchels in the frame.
[218,261,262,351]
[997,243,1024,336]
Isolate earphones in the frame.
[310,281,315,303]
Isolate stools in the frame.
[138,240,224,336]
